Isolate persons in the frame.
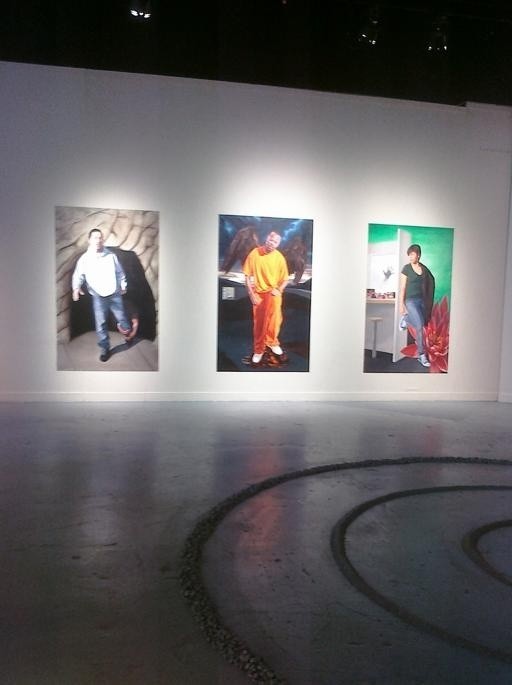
[242,230,289,368]
[115,298,141,344]
[399,244,434,368]
[71,228,133,362]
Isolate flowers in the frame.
[399,296,450,373]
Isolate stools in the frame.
[367,316,383,358]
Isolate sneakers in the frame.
[100,348,110,361]
[268,345,283,355]
[418,354,431,366]
[399,316,409,330]
[252,353,264,363]
